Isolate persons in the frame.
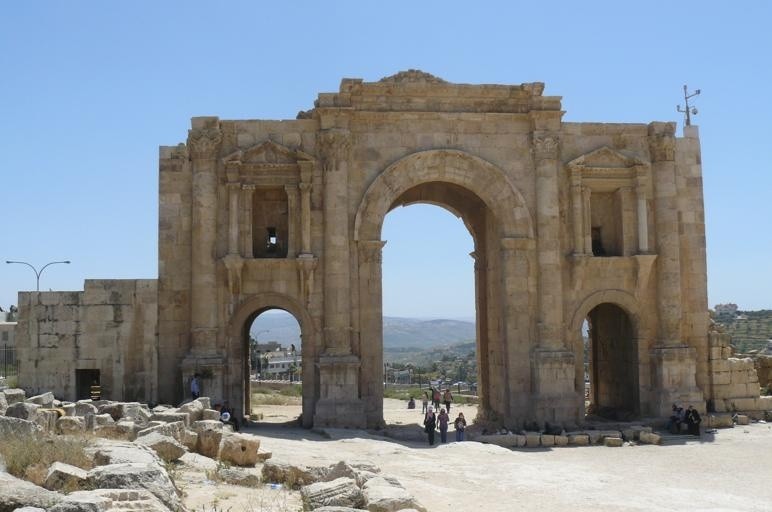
[669,401,701,437]
[214,400,240,432]
[407,384,455,414]
[190,372,201,401]
[423,406,467,447]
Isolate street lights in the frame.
[6,260,71,291]
